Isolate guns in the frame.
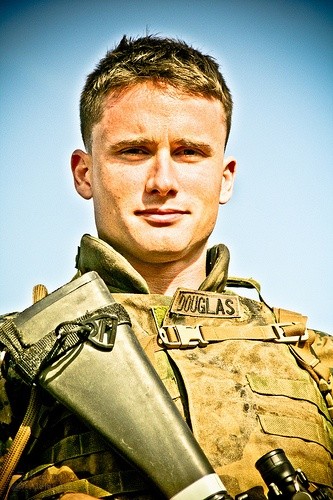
[1,270,333,500]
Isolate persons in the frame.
[0,24,332,499]
